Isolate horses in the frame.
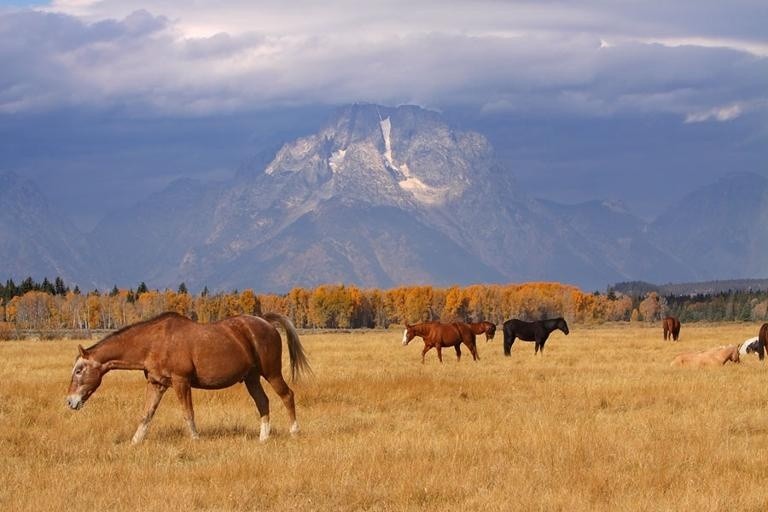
[670,345,740,370]
[401,320,481,363]
[502,317,570,358]
[739,336,766,357]
[662,317,681,342]
[467,320,496,342]
[758,323,768,360]
[65,310,318,446]
